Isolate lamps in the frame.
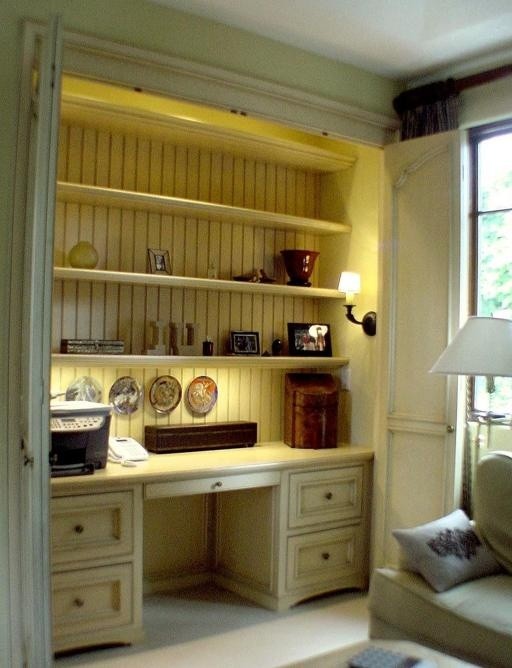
[338,272,376,336]
[429,316,512,394]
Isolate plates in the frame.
[65,375,217,414]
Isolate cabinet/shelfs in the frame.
[212,461,374,609]
[47,485,145,654]
[52,180,350,368]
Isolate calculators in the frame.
[346,644,437,668]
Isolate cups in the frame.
[203,341,214,356]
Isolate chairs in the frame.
[365,450,512,668]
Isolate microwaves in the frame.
[48,411,109,471]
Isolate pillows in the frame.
[392,509,495,592]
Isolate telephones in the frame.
[107,436,149,461]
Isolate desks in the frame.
[285,639,480,668]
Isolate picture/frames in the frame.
[288,322,332,357]
[230,331,259,355]
